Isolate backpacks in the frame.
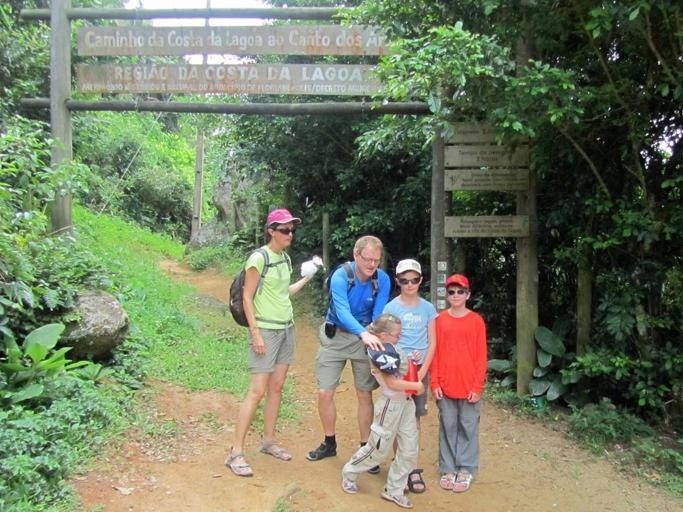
[327,261,380,300]
[228,249,292,326]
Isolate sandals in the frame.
[341,468,358,494]
[306,441,337,461]
[225,452,254,476]
[259,441,292,461]
[408,468,426,493]
[382,487,413,508]
[440,473,456,490]
[453,471,473,492]
[369,465,381,474]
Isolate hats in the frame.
[445,274,470,290]
[266,209,301,228]
[396,259,422,276]
[367,343,400,375]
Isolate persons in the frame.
[339,313,424,510]
[303,235,392,473]
[427,273,487,494]
[224,208,326,475]
[380,256,440,493]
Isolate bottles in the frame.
[401,352,418,395]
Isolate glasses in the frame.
[271,225,296,234]
[398,278,421,285]
[448,290,465,295]
[361,255,381,264]
